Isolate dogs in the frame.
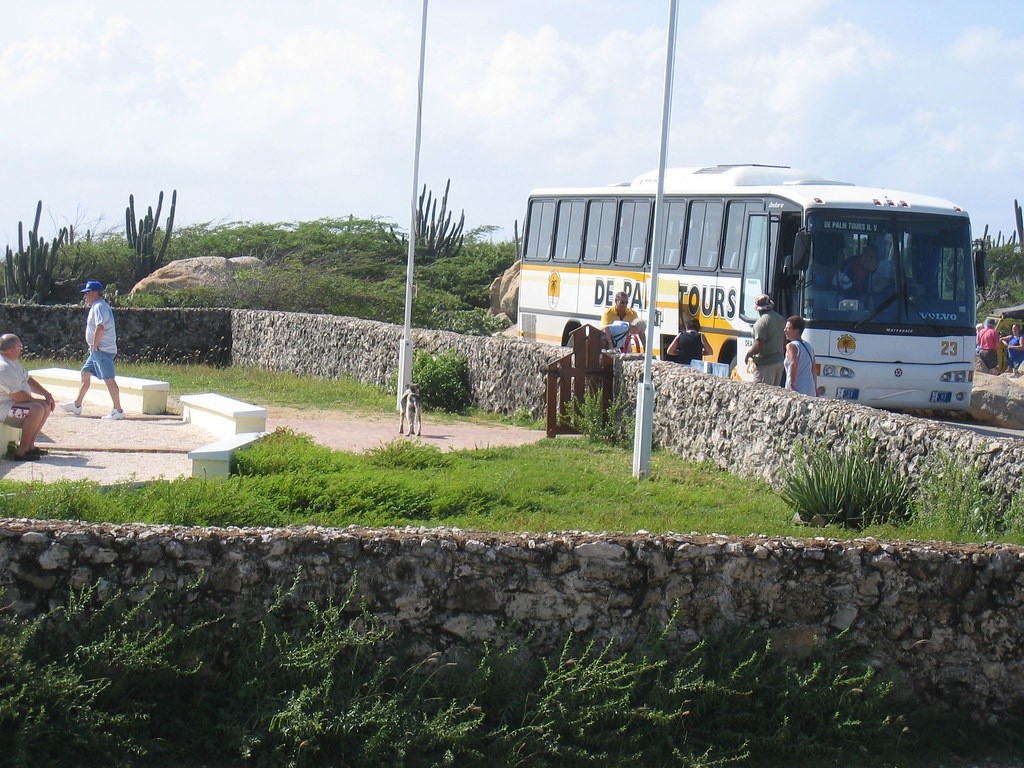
[398,383,421,437]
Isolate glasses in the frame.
[616,299,627,304]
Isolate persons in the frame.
[976,319,999,375]
[666,318,713,364]
[601,291,645,353]
[783,315,821,397]
[58,281,126,420]
[745,294,787,386]
[0,334,55,461]
[852,245,879,288]
[999,324,1024,372]
[603,318,647,349]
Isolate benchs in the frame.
[0,424,21,455]
[188,433,273,479]
[23,367,171,415]
[180,392,268,436]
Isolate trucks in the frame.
[983,303,1024,377]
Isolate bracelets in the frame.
[747,354,752,357]
[608,340,613,342]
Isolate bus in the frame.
[516,162,988,412]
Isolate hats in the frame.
[754,294,776,311]
[986,319,996,326]
[81,281,103,294]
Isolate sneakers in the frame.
[59,402,82,415]
[102,408,124,420]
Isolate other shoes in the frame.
[29,446,48,455]
[14,453,41,461]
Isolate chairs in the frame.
[545,243,760,269]
